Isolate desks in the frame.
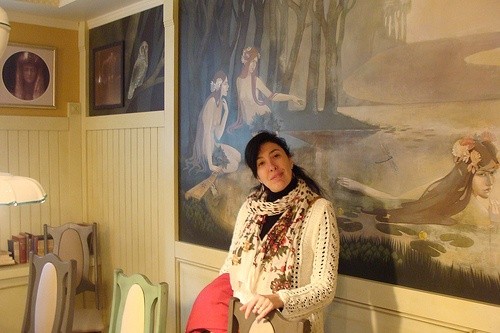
[0,263,33,333]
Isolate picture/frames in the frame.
[92,40,125,110]
[0,42,57,109]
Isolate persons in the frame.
[219,129,340,333]
[15,52,45,100]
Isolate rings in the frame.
[260,307,263,311]
[254,306,259,309]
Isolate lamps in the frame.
[0,172,48,204]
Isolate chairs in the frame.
[106,269,168,333]
[227,297,311,333]
[20,222,99,333]
